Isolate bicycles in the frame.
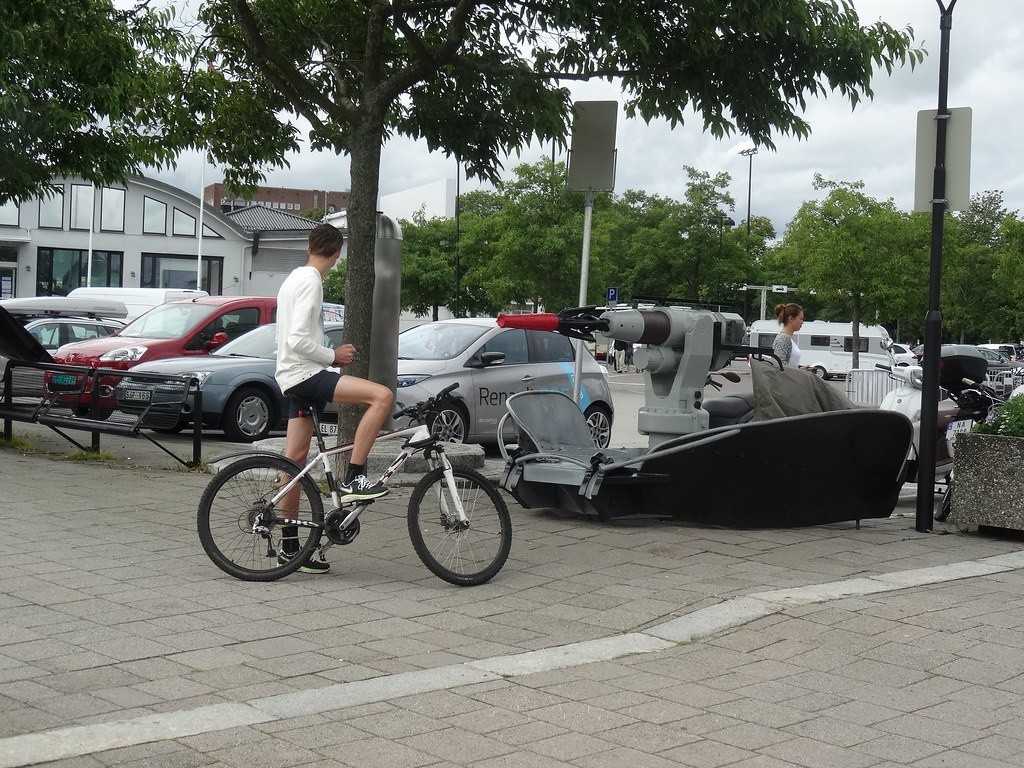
[195,381,514,588]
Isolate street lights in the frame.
[739,147,757,232]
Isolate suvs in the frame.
[43,293,345,421]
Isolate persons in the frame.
[609,339,633,373]
[772,303,810,371]
[274,224,394,573]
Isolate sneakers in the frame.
[340,475,390,504]
[277,549,330,573]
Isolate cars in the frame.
[316,316,614,456]
[0,285,212,400]
[581,306,1023,523]
[114,322,348,443]
